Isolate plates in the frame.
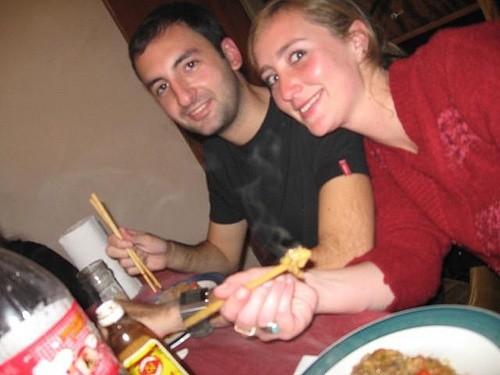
[151,273,231,327]
[300,304,500,375]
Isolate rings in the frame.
[257,321,281,335]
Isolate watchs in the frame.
[177,287,215,339]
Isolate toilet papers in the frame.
[56,214,143,301]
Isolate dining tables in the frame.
[84,269,396,375]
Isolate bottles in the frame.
[0,248,126,375]
[94,300,197,375]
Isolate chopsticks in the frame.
[183,261,285,329]
[89,193,161,294]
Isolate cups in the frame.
[77,260,129,304]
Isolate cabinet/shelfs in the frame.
[239,0,500,67]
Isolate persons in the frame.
[105,4,376,338]
[213,1,499,342]
